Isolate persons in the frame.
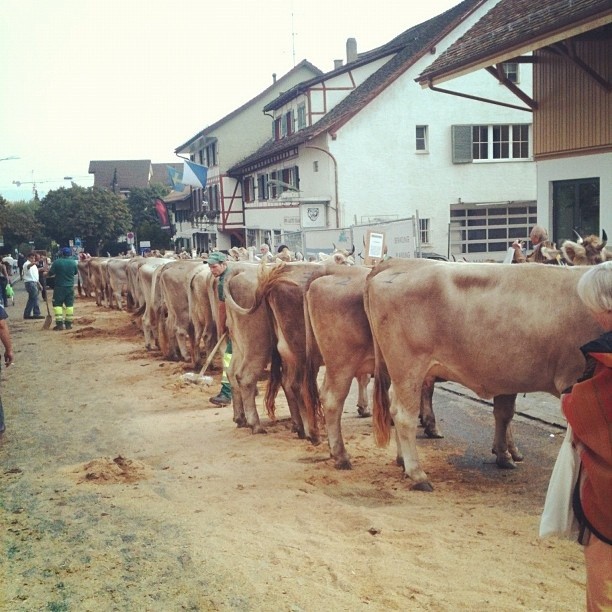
[17,253,27,280]
[0,257,8,307]
[0,305,14,434]
[260,244,273,263]
[22,253,45,319]
[43,247,78,330]
[559,261,612,612]
[203,252,259,405]
[278,245,289,253]
[35,254,47,301]
[512,226,549,263]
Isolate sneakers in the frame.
[210,393,231,405]
[53,325,63,330]
[65,322,72,329]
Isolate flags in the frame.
[168,167,186,193]
[182,160,208,190]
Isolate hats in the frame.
[60,247,72,255]
[202,253,228,263]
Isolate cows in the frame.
[98,260,109,308]
[221,243,365,435]
[299,263,524,469]
[362,255,612,492]
[125,256,139,307]
[106,258,130,310]
[160,259,205,362]
[139,257,177,350]
[77,258,92,298]
[186,261,235,370]
[87,256,103,307]
[264,262,395,446]
[541,228,608,265]
